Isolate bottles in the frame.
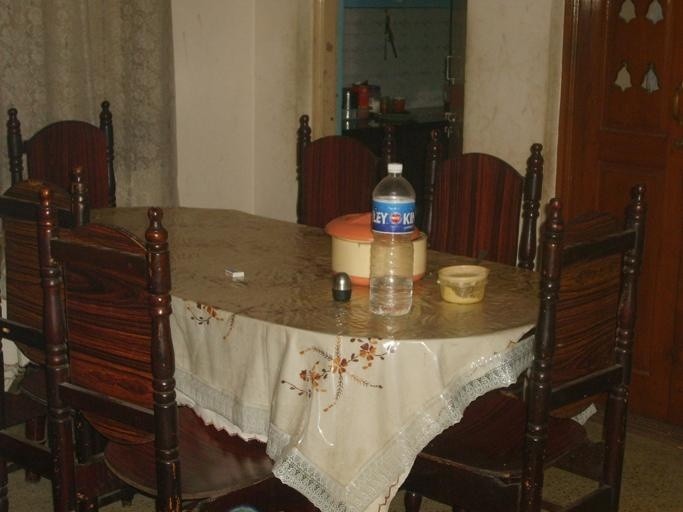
[343,79,407,124]
[366,159,417,318]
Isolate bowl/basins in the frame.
[437,265,489,304]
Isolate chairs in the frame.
[294,115,396,230]
[426,129,543,419]
[0,169,136,508]
[6,99,119,216]
[40,191,311,510]
[398,186,647,512]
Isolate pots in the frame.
[324,212,428,288]
